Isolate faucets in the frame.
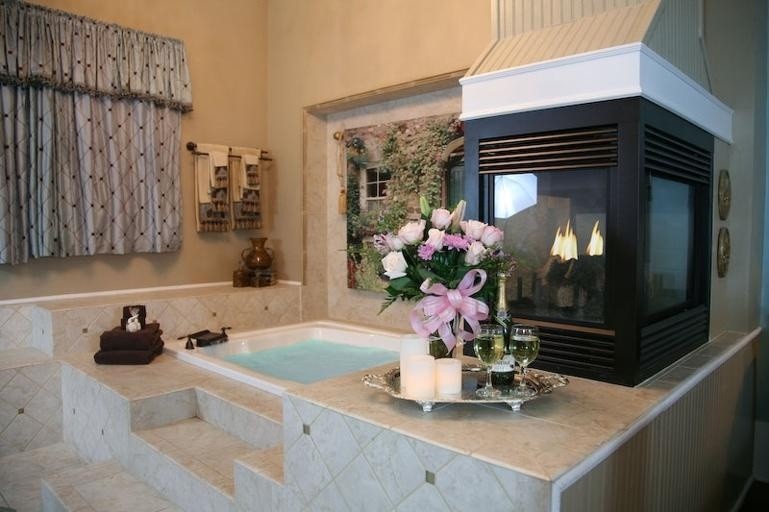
[192,330,229,346]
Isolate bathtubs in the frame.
[164,321,402,395]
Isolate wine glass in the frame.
[474,324,541,397]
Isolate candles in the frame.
[398,333,462,398]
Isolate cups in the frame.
[400,334,463,398]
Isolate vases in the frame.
[426,317,458,358]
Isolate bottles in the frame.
[486,272,516,385]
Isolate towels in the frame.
[94,323,165,365]
[194,143,264,234]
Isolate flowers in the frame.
[337,196,514,358]
